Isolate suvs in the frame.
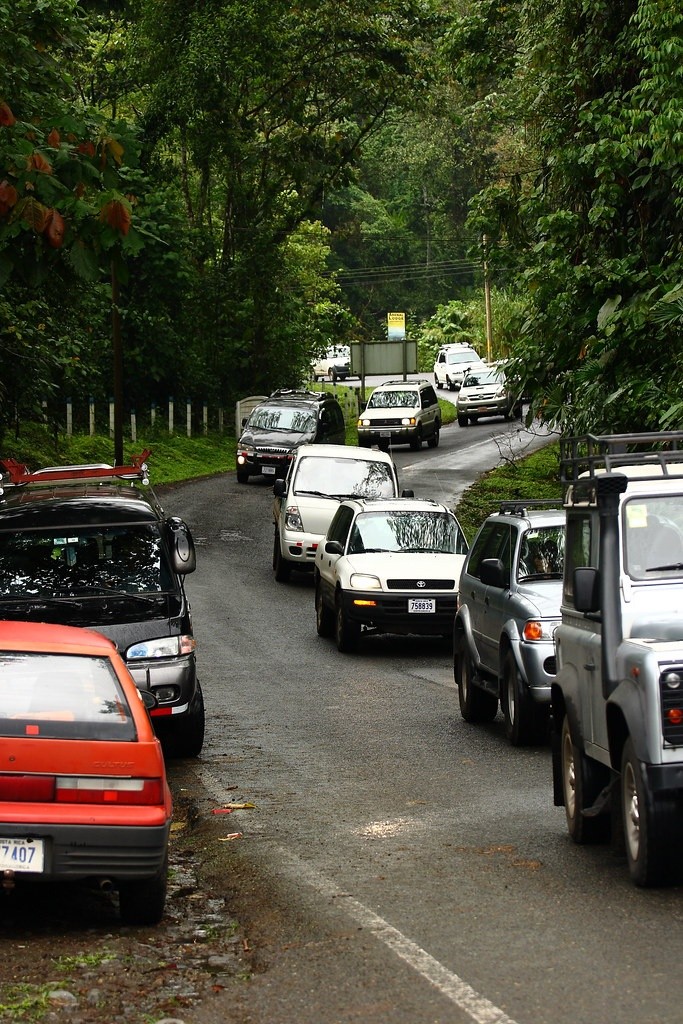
[432,341,487,390]
[0,454,204,761]
[550,425,683,889]
[457,364,523,426]
[356,378,441,451]
[306,344,361,380]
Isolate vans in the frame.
[236,387,346,486]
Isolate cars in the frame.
[0,620,172,926]
[272,441,415,585]
[314,490,474,657]
[450,505,570,745]
